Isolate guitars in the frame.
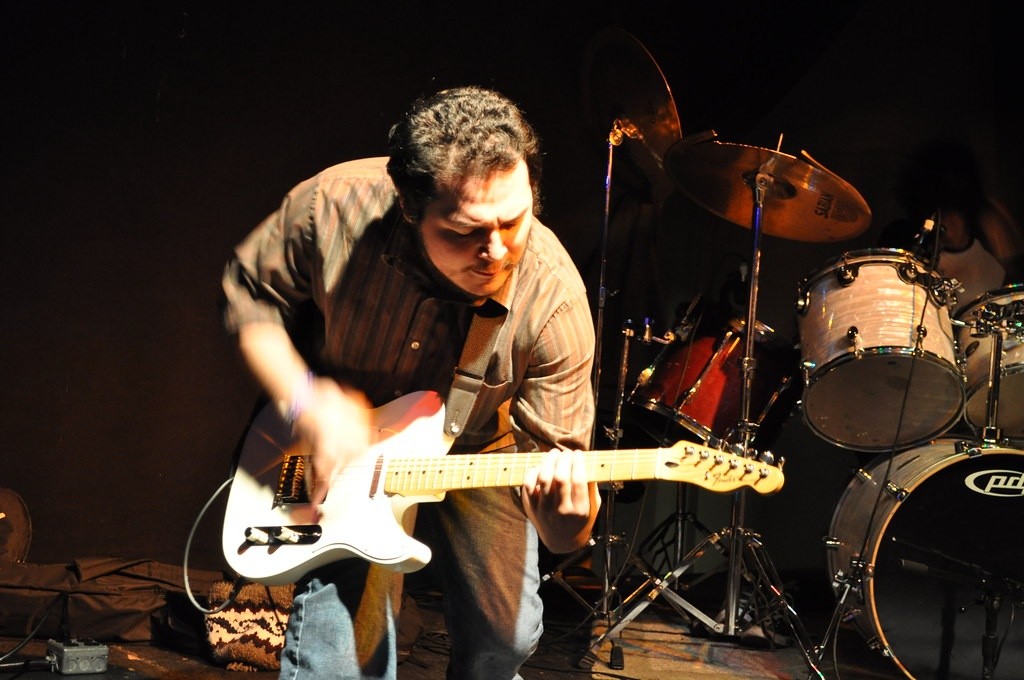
[222,390,784,585]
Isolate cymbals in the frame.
[665,129,872,242]
[602,24,682,162]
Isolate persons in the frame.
[217,87,603,680]
[881,140,1024,288]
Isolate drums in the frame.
[828,437,1024,680]
[627,293,802,447]
[796,247,967,453]
[949,283,1024,450]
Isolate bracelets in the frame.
[283,399,309,426]
